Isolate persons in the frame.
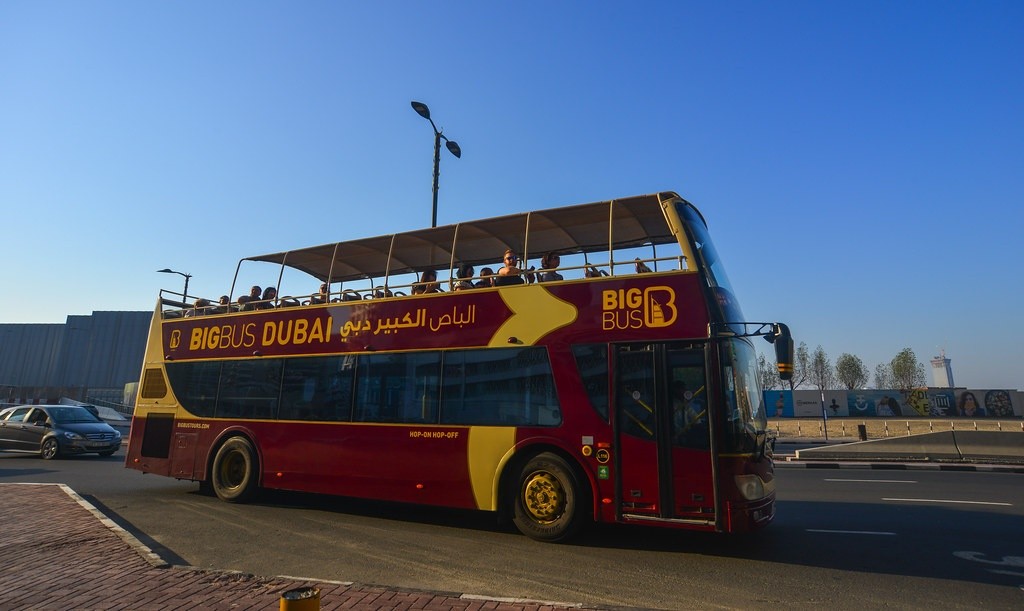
[453,249,524,292]
[311,284,330,305]
[671,380,702,447]
[830,399,840,408]
[184,286,276,318]
[959,391,985,416]
[541,251,563,282]
[414,268,440,295]
[876,396,902,416]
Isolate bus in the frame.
[120,188,797,545]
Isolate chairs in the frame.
[163,256,651,318]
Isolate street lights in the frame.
[155,268,192,310]
[411,98,467,264]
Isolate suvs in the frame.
[0,404,124,460]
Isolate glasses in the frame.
[550,257,560,260]
[505,255,517,260]
